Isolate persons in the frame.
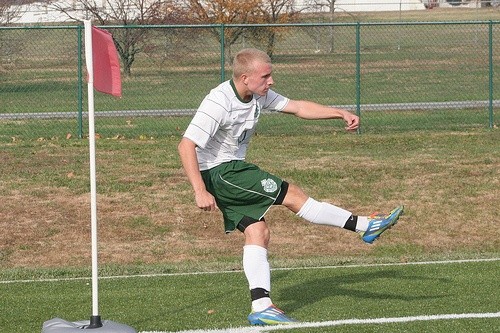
[177,49,405,325]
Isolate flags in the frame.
[86,24,122,96]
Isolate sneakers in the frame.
[358,204,405,244]
[247,304,301,327]
